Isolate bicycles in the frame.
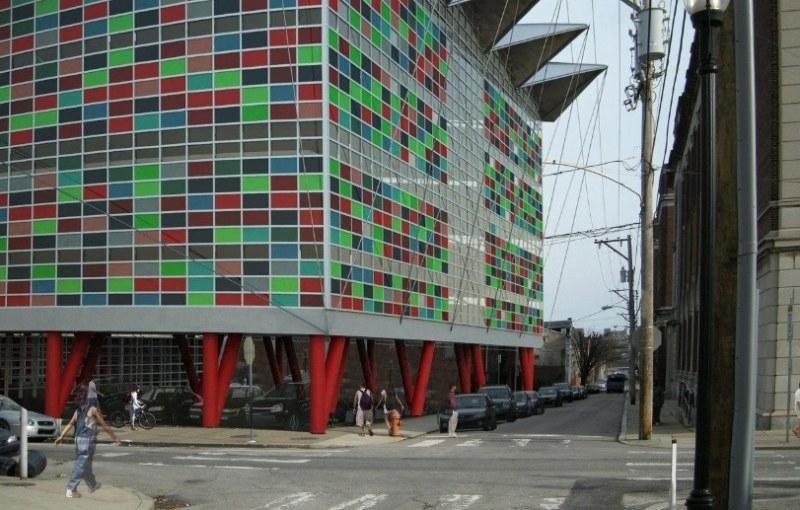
[111,404,156,429]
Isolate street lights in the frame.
[683,0,730,510]
[602,305,636,405]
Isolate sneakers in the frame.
[358,430,373,435]
[89,482,101,493]
[132,428,137,430]
[66,488,81,498]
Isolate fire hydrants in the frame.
[389,409,401,436]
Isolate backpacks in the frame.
[359,388,371,410]
[386,390,396,410]
[124,392,134,411]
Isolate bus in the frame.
[607,374,625,393]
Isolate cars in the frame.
[538,382,601,407]
[478,384,544,422]
[440,393,496,433]
[0,394,58,477]
[141,377,311,430]
